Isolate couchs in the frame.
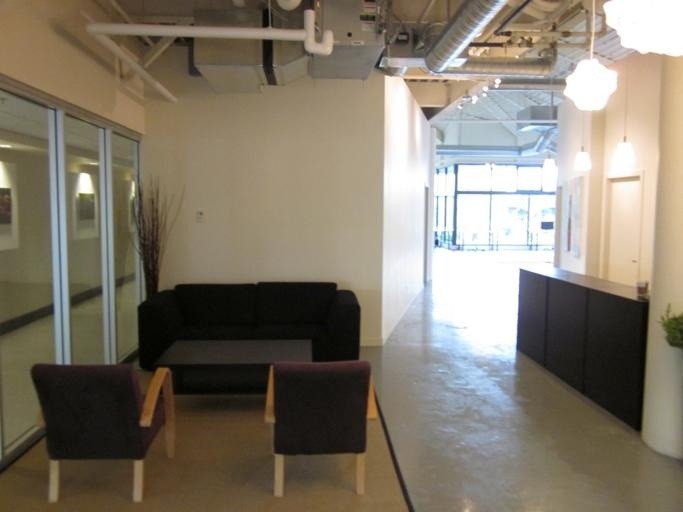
[138,282,361,395]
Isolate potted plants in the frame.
[641,302,683,461]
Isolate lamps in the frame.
[544,0,641,179]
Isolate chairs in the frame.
[31,363,177,503]
[264,360,378,497]
